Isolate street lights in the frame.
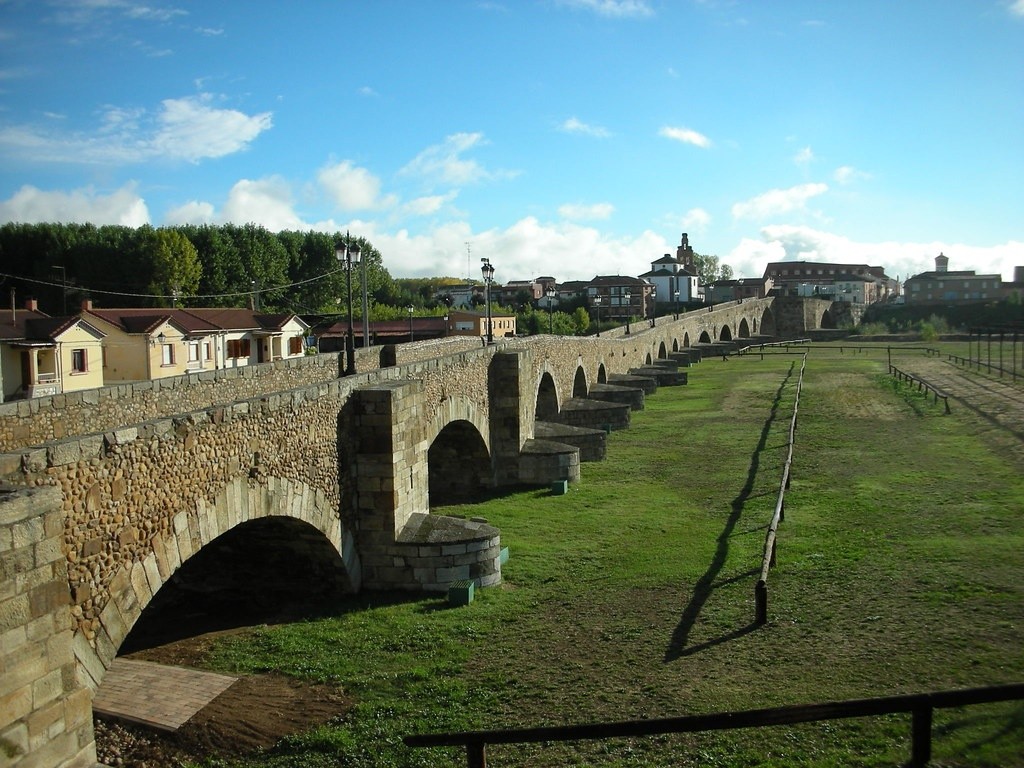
[674,290,680,320]
[172,299,177,308]
[545,286,556,334]
[332,229,363,375]
[650,290,657,328]
[407,304,414,342]
[624,290,631,334]
[479,257,495,346]
[443,313,449,337]
[707,284,715,312]
[50,265,67,316]
[593,294,602,337]
[802,282,807,296]
[739,279,744,303]
